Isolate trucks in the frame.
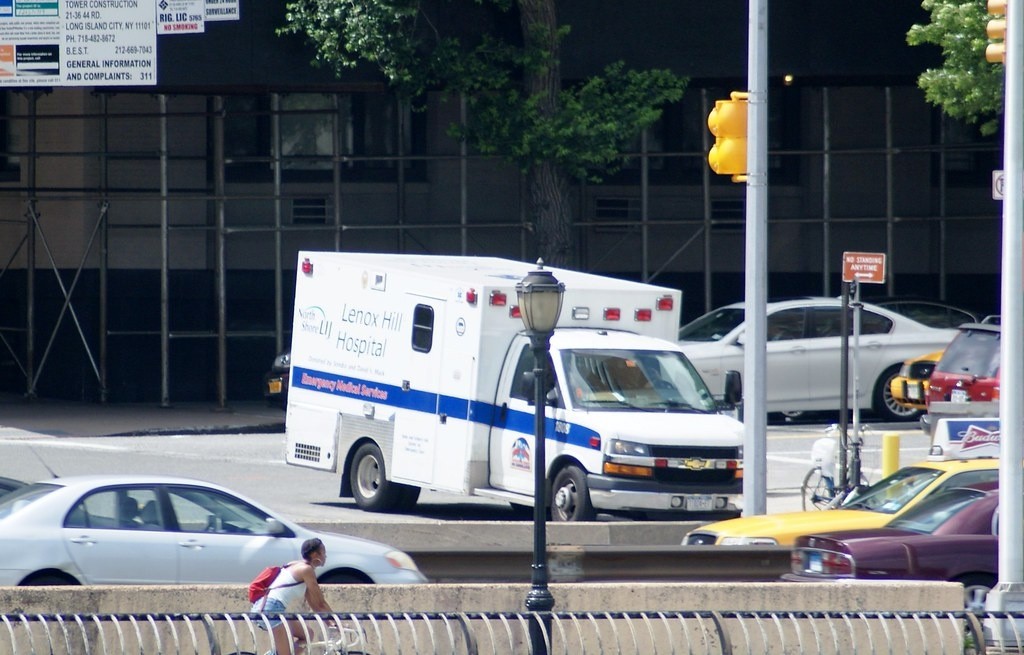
[282,248,746,527]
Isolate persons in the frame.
[248,538,338,655]
[619,359,653,398]
[815,313,841,336]
[768,314,795,341]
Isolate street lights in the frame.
[515,255,567,655]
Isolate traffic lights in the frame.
[985,0,1007,65]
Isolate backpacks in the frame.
[248,563,305,613]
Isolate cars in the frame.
[679,293,965,423]
[1,475,434,589]
[777,467,1001,637]
[868,300,984,334]
[263,347,291,410]
[676,456,1002,549]
[918,314,1003,434]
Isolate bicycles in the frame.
[223,618,374,655]
[800,422,871,517]
